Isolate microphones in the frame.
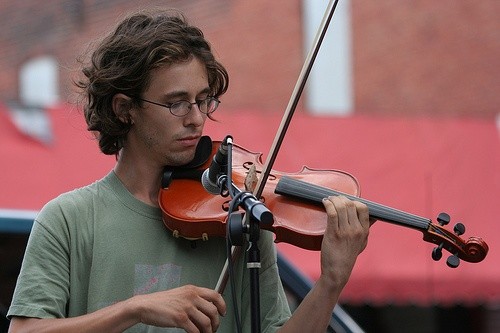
[201,135,231,193]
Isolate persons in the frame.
[6,10,378,333]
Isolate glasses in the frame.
[131,92,222,117]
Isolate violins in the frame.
[157,138,489,269]
